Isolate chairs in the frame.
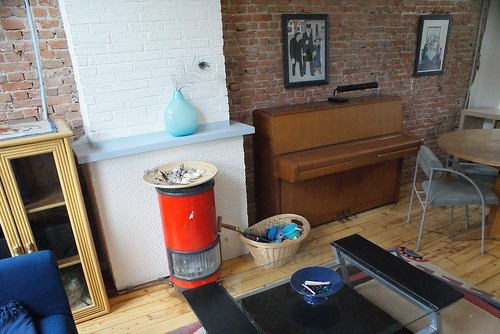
[406,146,499,255]
[0,250,78,334]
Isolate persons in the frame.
[290,24,322,76]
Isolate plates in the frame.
[143,160,217,188]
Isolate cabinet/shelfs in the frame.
[450,107,500,176]
[0,120,111,325]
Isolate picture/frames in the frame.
[413,15,452,78]
[281,13,329,89]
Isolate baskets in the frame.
[239,214,309,270]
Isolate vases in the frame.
[164,90,197,137]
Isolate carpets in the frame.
[165,245,500,334]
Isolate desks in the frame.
[438,128,500,239]
[180,231,464,334]
